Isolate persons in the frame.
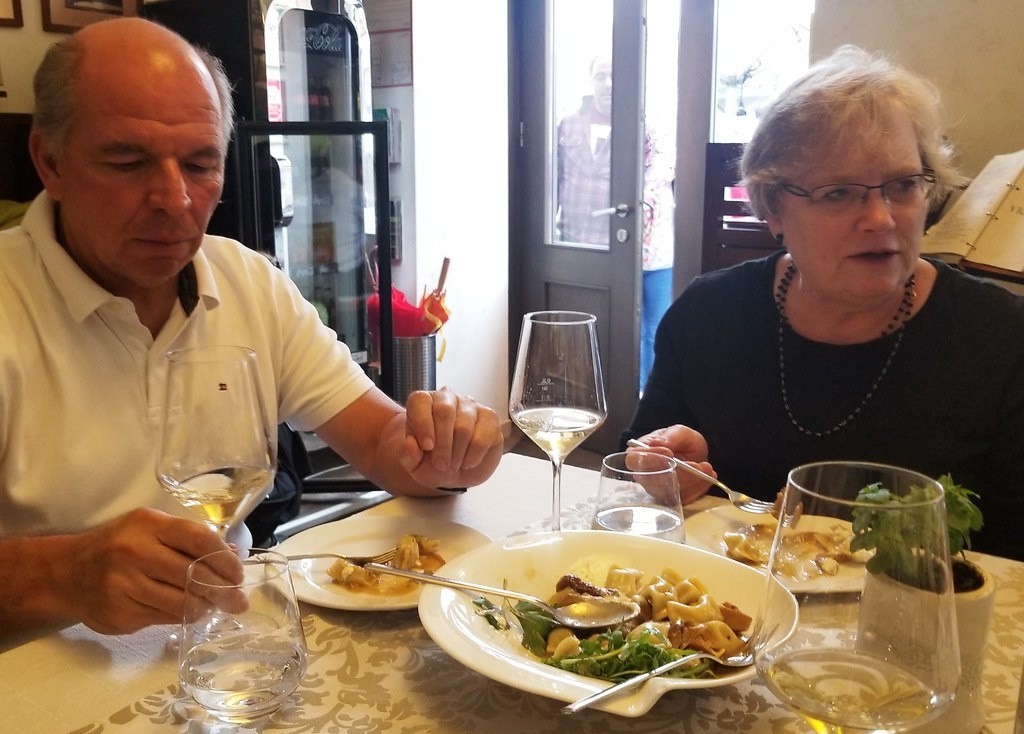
[0,16,504,637]
[618,43,1024,565]
[293,139,375,296]
[557,52,675,394]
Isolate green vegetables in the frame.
[478,580,720,684]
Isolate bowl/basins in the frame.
[417,529,799,715]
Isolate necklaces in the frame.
[774,262,914,438]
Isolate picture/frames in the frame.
[40,0,142,37]
[0,0,24,28]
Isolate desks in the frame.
[0,452,1024,734]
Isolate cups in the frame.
[593,451,687,545]
[180,549,309,724]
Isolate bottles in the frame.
[307,76,318,122]
[318,76,332,123]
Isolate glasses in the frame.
[782,166,936,211]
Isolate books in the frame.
[919,150,1024,278]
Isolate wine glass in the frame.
[751,459,960,734]
[155,344,278,659]
[509,310,607,534]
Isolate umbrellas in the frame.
[364,246,452,338]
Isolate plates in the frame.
[266,516,494,611]
[685,503,876,593]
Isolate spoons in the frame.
[364,562,640,629]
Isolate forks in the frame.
[239,548,398,570]
[627,438,775,514]
[562,620,779,715]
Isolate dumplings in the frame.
[725,491,802,561]
[327,534,442,585]
[544,556,742,660]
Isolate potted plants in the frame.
[849,476,990,734]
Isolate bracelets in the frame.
[433,487,468,496]
